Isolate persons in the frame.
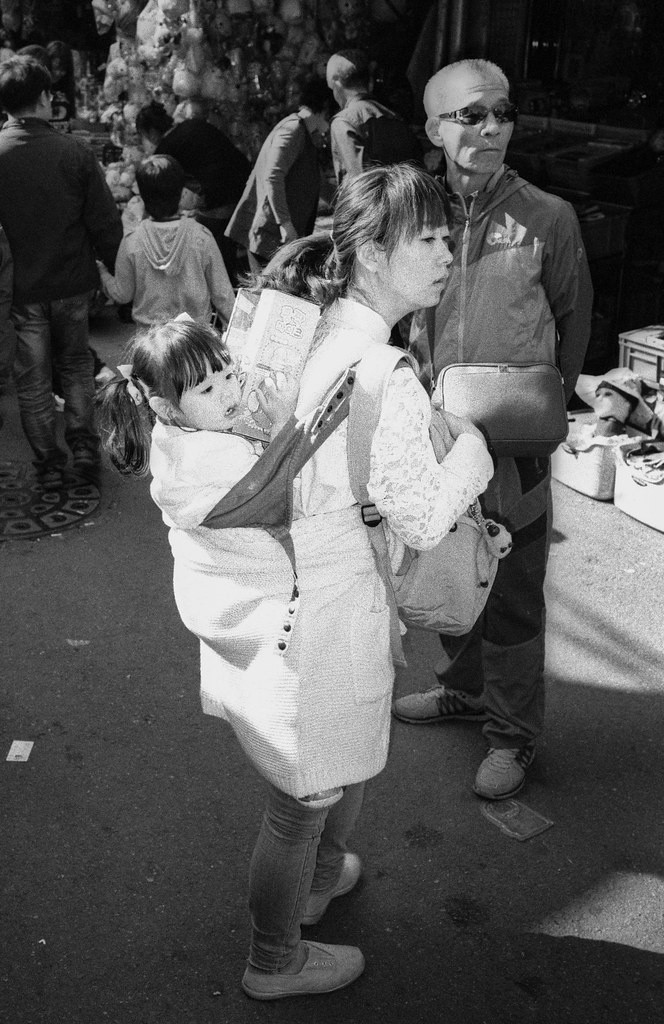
[574,366,654,438]
[393,60,591,800]
[0,0,593,1000]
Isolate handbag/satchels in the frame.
[430,361,569,455]
[391,515,514,637]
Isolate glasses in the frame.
[436,104,519,126]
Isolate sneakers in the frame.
[73,444,100,467]
[473,738,539,799]
[391,686,493,726]
[41,472,65,489]
[241,851,365,999]
